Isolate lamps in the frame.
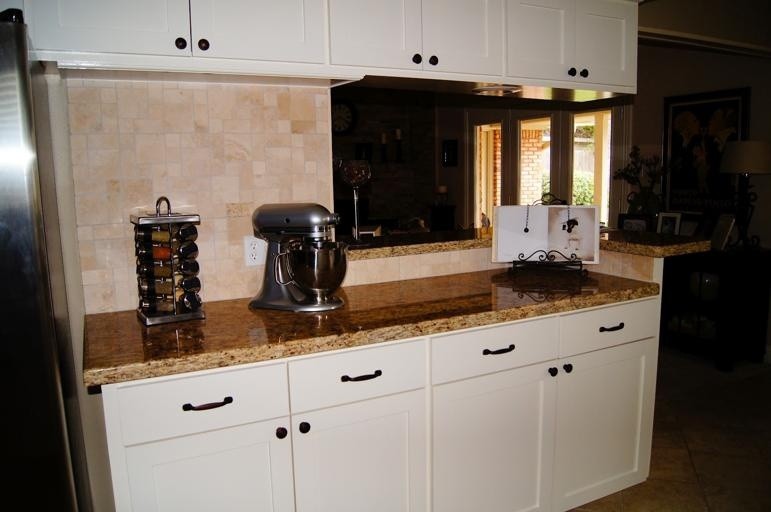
[720,139,771,250]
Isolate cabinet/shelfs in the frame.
[328,0,505,89]
[430,285,660,512]
[24,1,328,87]
[83,334,429,512]
[505,0,639,94]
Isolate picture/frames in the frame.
[657,213,681,234]
[661,87,750,218]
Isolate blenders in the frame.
[250,304,355,348]
[243,200,347,311]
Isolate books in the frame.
[491,205,600,263]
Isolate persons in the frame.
[672,122,738,222]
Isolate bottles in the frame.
[141,326,204,371]
[137,224,205,311]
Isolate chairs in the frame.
[711,216,735,254]
